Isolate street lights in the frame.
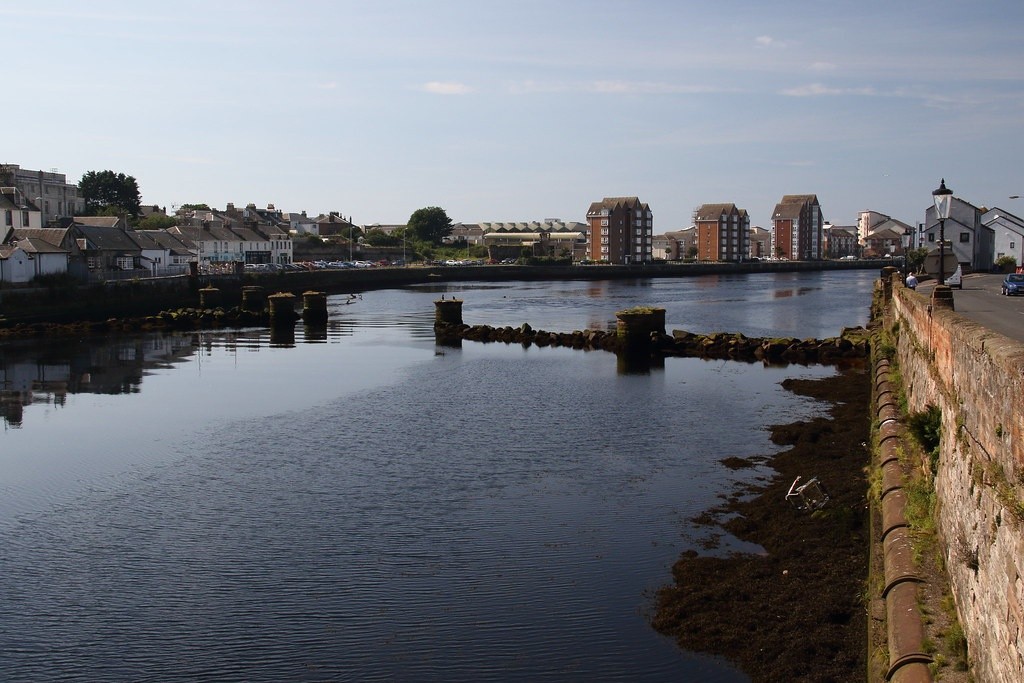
[403,228,409,262]
[900,228,910,287]
[931,177,953,287]
[889,242,896,265]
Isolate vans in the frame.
[941,263,962,290]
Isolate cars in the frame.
[1001,274,1024,296]
[752,256,790,262]
[580,260,592,265]
[841,254,881,260]
[242,259,404,272]
[424,258,518,265]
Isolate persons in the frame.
[906,271,918,291]
